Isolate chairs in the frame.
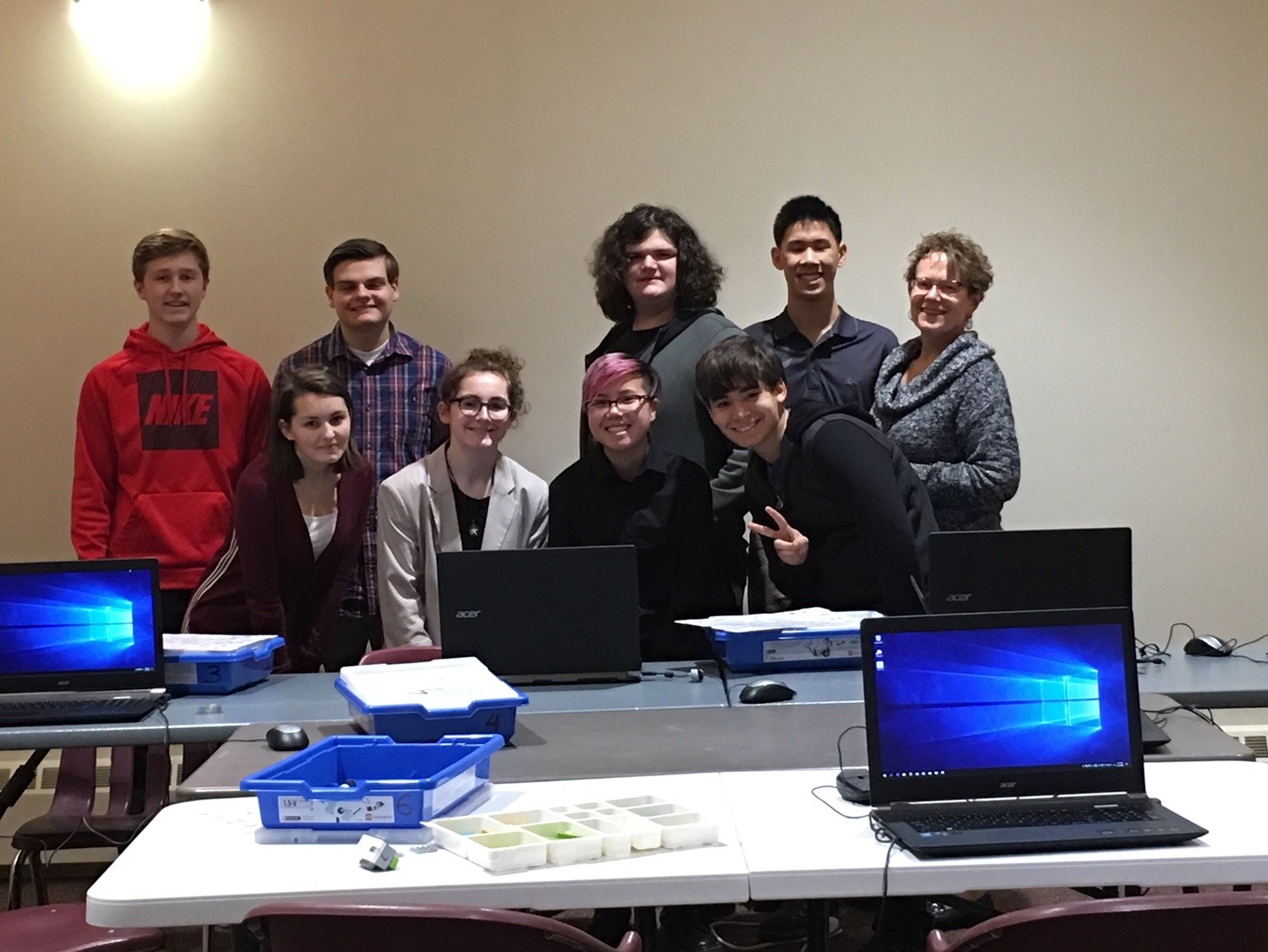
[0,596,1267,952]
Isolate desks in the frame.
[175,694,1256,800]
[721,652,1268,707]
[741,756,1268,952]
[84,773,751,952]
[1,644,728,747]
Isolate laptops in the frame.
[0,558,166,728]
[859,527,1208,858]
[436,544,642,687]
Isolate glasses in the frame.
[449,396,514,421]
[909,276,965,295]
[585,395,653,417]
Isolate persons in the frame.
[579,201,750,615]
[234,362,375,676]
[870,228,1022,531]
[71,227,272,816]
[694,333,942,942]
[269,236,456,674]
[375,346,549,647]
[549,352,737,947]
[740,195,899,429]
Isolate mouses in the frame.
[267,723,309,750]
[1184,634,1232,657]
[739,679,797,703]
[836,768,872,804]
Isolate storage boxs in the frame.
[333,650,527,752]
[705,606,882,669]
[240,729,508,826]
[160,634,287,695]
